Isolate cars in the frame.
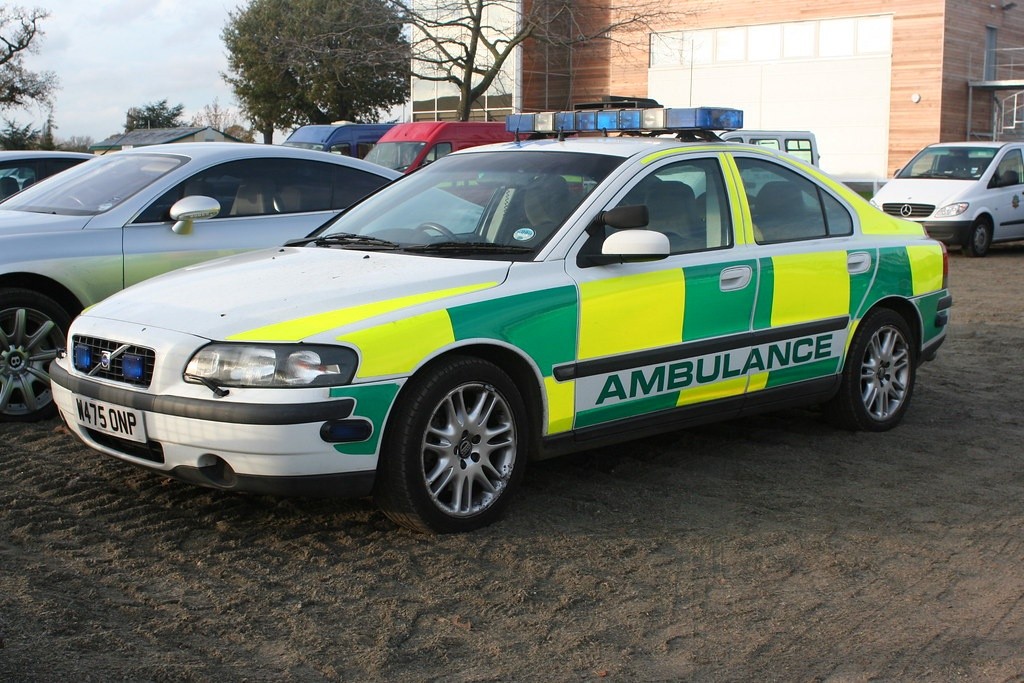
[1,150,100,211]
[868,141,1024,258]
[1,141,486,424]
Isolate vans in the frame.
[265,120,820,211]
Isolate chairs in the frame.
[749,180,821,240]
[975,162,1001,189]
[0,177,20,202]
[184,175,385,217]
[648,182,707,251]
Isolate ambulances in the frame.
[48,95,954,537]
